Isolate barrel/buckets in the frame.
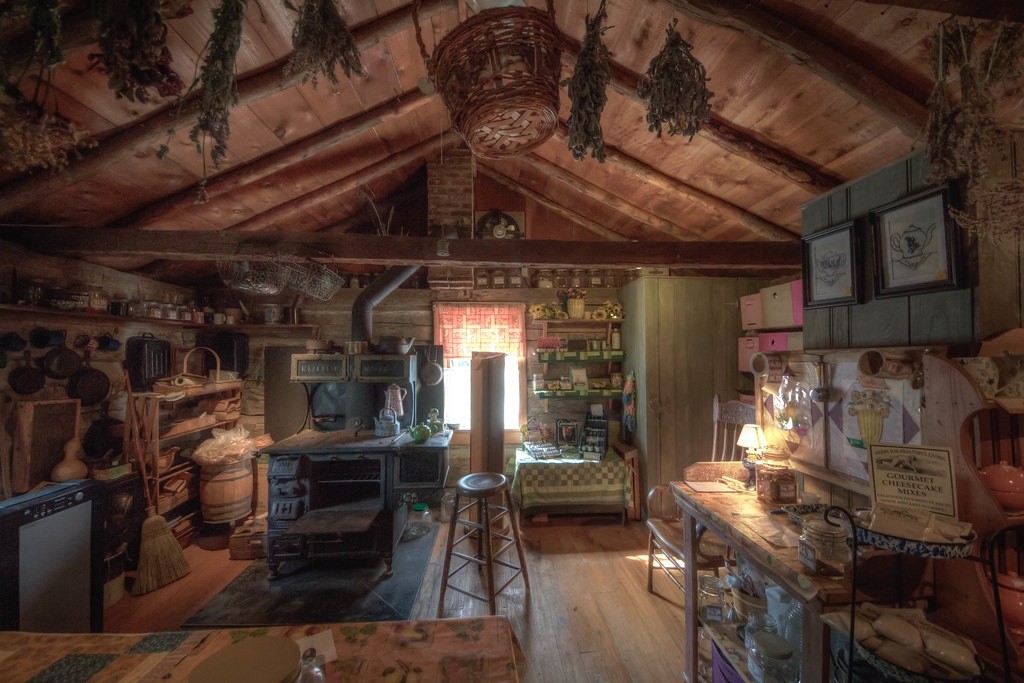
[199,459,253,524]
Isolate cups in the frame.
[799,492,821,505]
[284,308,300,324]
[226,315,234,325]
[213,313,226,324]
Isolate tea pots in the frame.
[372,407,400,437]
[407,422,438,443]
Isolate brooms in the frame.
[124,377,193,597]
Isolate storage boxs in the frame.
[737,277,802,404]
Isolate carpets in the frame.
[182,520,440,628]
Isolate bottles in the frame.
[441,492,461,522]
[532,373,545,391]
[264,303,280,325]
[797,521,850,578]
[755,444,798,505]
[475,268,604,288]
[697,573,800,683]
[408,503,433,535]
[22,278,213,323]
[611,373,622,388]
[340,272,381,288]
[611,328,621,351]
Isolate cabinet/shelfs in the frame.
[531,316,626,397]
[2,304,321,583]
[668,325,1023,683]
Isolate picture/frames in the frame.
[870,179,956,299]
[802,213,864,309]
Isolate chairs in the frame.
[646,392,766,618]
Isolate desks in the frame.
[506,447,631,528]
[1,614,528,682]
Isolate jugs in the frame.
[384,383,407,420]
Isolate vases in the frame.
[568,299,583,320]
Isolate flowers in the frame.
[528,287,626,322]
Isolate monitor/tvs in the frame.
[0,477,102,635]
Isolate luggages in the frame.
[126,333,171,392]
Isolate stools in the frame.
[436,472,531,614]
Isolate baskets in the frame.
[215,241,345,301]
[412,0,563,158]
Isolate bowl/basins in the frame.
[447,424,460,429]
[730,586,768,623]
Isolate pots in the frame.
[419,346,443,386]
[9,330,128,458]
